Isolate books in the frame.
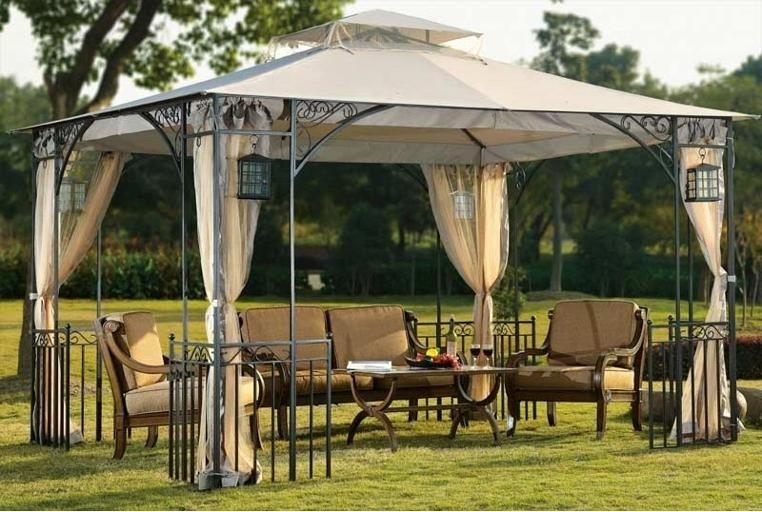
[347,360,392,373]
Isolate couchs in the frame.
[88,306,268,456]
[504,297,651,438]
[236,302,468,444]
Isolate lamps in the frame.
[53,169,90,218]
[449,181,477,223]
[681,146,722,204]
[233,136,274,201]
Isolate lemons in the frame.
[426,349,438,356]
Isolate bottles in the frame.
[445,315,457,356]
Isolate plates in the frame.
[402,354,457,367]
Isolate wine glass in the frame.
[482,344,493,369]
[469,343,481,368]
